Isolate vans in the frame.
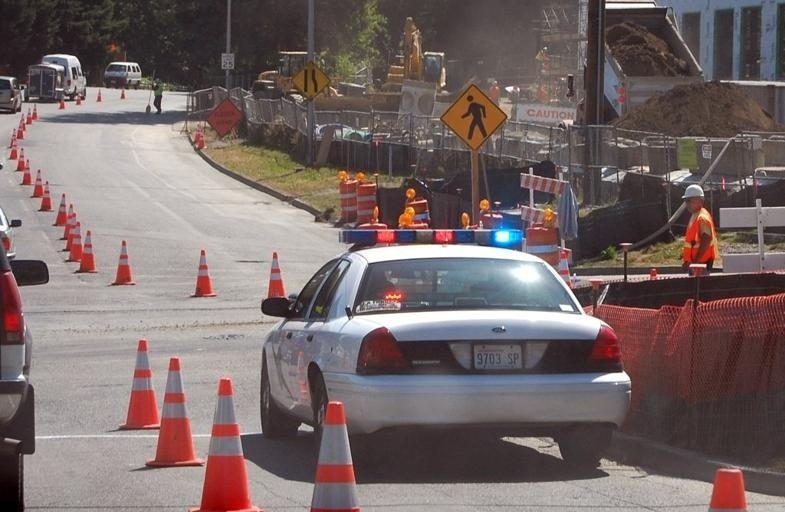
[104,61,144,90]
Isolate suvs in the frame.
[1,237,48,512]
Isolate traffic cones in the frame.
[59,96,66,108]
[76,91,82,105]
[119,85,127,99]
[264,252,285,299]
[145,357,206,470]
[194,377,259,511]
[108,238,137,287]
[193,124,209,150]
[301,401,366,512]
[189,249,219,297]
[706,464,751,512]
[649,268,658,280]
[8,102,98,273]
[96,89,103,102]
[110,336,161,432]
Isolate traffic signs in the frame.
[221,52,236,71]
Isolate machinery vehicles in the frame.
[370,18,452,100]
[257,47,338,107]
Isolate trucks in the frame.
[41,52,88,99]
[566,0,708,133]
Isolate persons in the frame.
[682,185,716,275]
[490,81,501,106]
[535,47,556,101]
[152,79,162,114]
[512,81,519,103]
[429,60,437,74]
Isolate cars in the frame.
[0,162,21,266]
[0,75,24,113]
[260,227,635,469]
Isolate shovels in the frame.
[146,70,156,113]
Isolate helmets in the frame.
[681,184,704,199]
